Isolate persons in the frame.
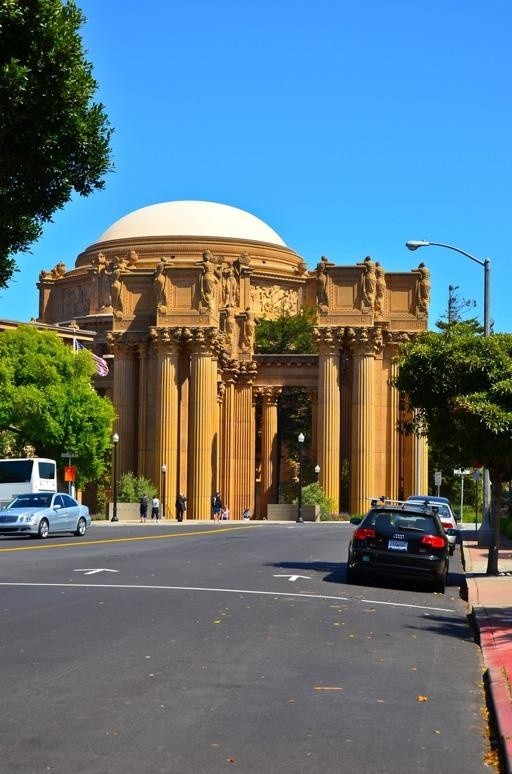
[211,491,224,525]
[223,506,230,520]
[111,248,239,311]
[138,493,148,523]
[316,256,431,313]
[151,495,160,519]
[176,493,185,522]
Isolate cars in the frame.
[348,496,458,593]
[1,493,91,539]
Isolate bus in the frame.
[0,458,58,509]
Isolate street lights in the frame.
[161,464,166,517]
[406,240,492,532]
[315,465,320,482]
[296,432,304,521]
[111,433,119,522]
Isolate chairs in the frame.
[374,514,430,530]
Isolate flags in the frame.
[76,340,109,377]
